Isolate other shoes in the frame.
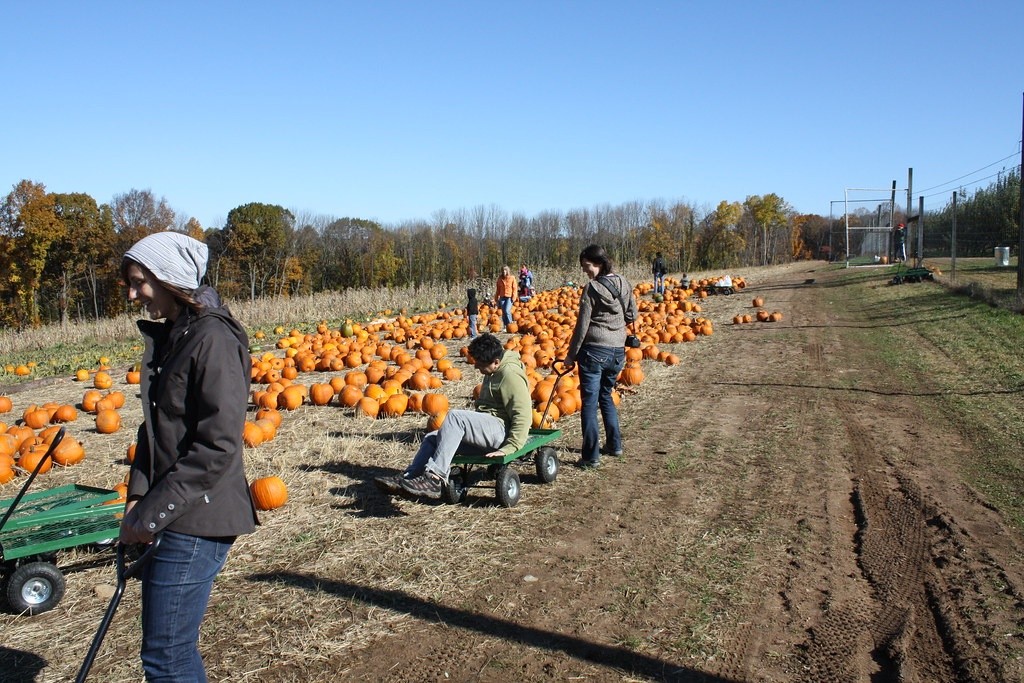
[468,335,477,341]
[573,458,602,470]
[599,444,622,457]
[502,328,507,333]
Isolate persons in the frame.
[680,273,688,290]
[119,231,263,683]
[564,245,638,468]
[652,252,667,295]
[495,264,518,329]
[466,288,479,340]
[894,223,906,262]
[518,264,535,303]
[374,332,534,499]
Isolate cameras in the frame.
[625,335,640,348]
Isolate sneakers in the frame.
[376,471,412,494]
[401,471,441,499]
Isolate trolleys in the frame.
[442,359,575,508]
[0,426,129,617]
[704,285,734,295]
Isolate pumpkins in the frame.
[242,303,475,509]
[0,354,142,520]
[476,285,581,430]
[612,277,782,405]
[880,256,888,264]
